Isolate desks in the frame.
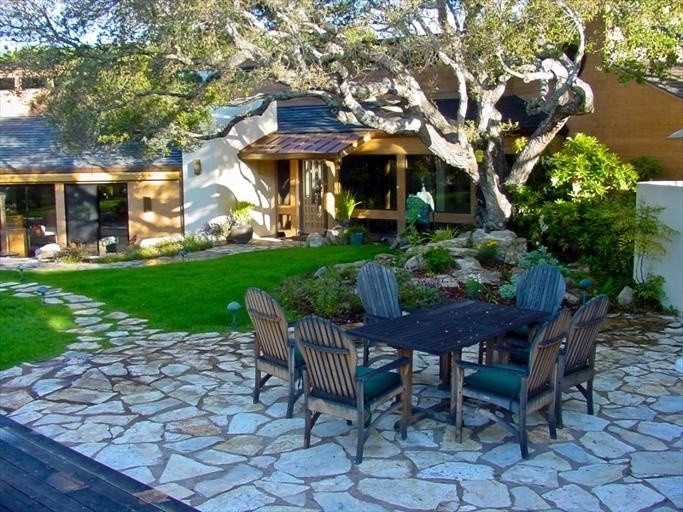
[346,300,552,443]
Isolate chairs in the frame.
[455,309,570,459]
[558,295,609,424]
[295,316,411,464]
[479,266,566,366]
[358,263,402,323]
[245,288,304,419]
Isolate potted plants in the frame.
[334,189,366,245]
[232,202,255,244]
